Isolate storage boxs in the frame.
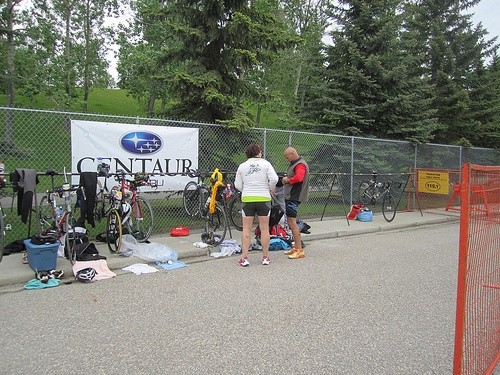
[23,239,60,273]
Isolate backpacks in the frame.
[64,227,107,260]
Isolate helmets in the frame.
[76,268,97,282]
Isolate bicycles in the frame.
[358,170,402,222]
[38,168,154,266]
[183,168,243,246]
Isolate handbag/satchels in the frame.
[255,223,292,251]
[170,225,190,236]
[347,203,373,222]
[269,203,284,225]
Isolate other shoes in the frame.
[36,271,48,283]
[48,269,65,279]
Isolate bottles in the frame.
[0,162,4,177]
[205,194,212,208]
[116,191,123,206]
[63,182,70,197]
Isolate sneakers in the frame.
[262,256,272,265]
[285,248,295,254]
[236,257,250,266]
[289,249,305,258]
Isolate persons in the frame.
[235,144,278,267]
[282,147,309,259]
